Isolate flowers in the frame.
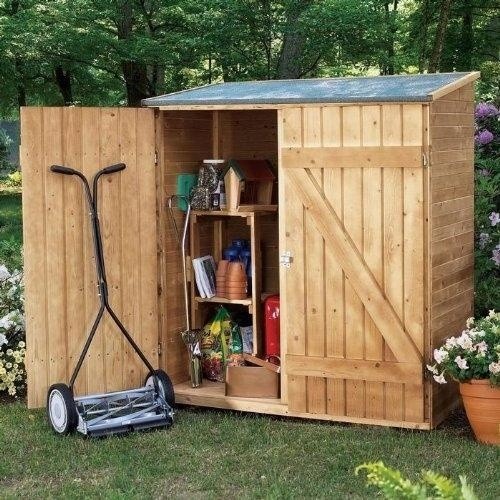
[0,266,26,397]
[425,102,500,386]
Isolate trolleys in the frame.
[45,159,176,436]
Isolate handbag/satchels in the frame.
[225,352,281,398]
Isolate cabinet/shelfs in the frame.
[184,209,276,357]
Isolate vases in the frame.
[460,379,500,444]
